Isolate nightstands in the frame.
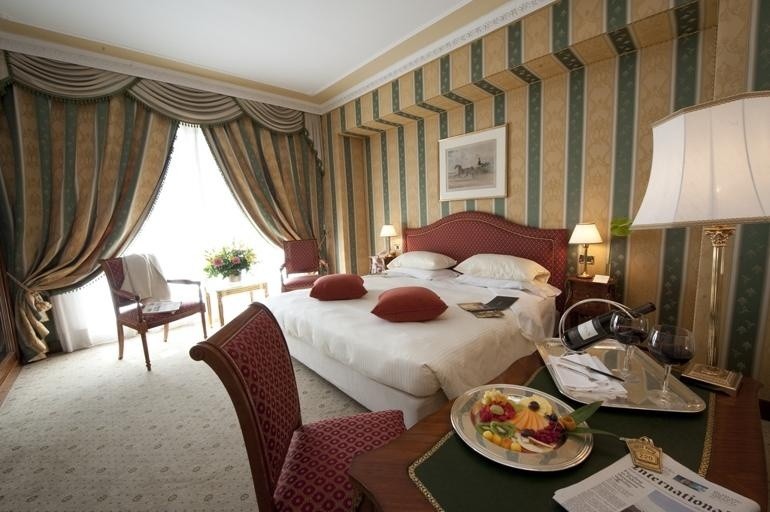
[369,254,398,274]
[568,274,616,317]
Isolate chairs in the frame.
[189,301,409,512]
[97,250,208,371]
[280,239,329,293]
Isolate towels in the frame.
[549,354,628,401]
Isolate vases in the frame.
[228,272,241,282]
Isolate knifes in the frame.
[560,357,625,382]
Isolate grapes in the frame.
[550,414,558,422]
[523,429,534,438]
[528,401,539,411]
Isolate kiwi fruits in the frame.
[490,422,512,439]
[476,423,491,434]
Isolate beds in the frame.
[261,211,568,430]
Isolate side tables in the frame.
[204,280,270,327]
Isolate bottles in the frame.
[562,301,657,350]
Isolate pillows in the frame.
[387,251,457,270]
[383,267,460,280]
[369,286,449,322]
[454,273,562,298]
[453,254,550,285]
[310,274,368,300]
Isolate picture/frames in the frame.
[436,123,509,202]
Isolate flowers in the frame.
[203,241,258,280]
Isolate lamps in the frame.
[380,225,397,255]
[628,90,770,398]
[568,222,604,279]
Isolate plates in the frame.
[533,337,707,413]
[450,383,593,472]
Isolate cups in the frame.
[652,326,695,409]
[609,309,648,379]
